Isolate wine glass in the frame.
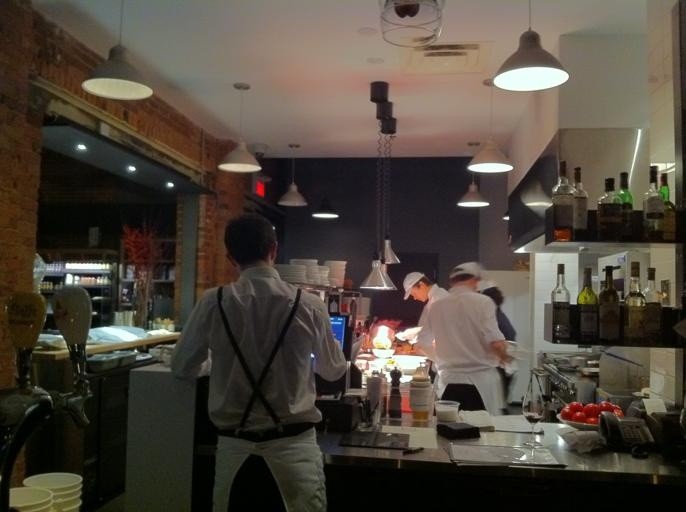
[522,391,546,448]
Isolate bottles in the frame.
[367,368,386,420]
[550,159,677,243]
[147,300,153,329]
[40,276,109,291]
[388,367,402,417]
[350,297,357,318]
[409,362,432,422]
[45,263,111,272]
[552,263,673,343]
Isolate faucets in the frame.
[61,389,93,430]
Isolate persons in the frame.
[403,262,517,414]
[171,213,347,512]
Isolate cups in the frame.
[6,473,84,512]
[434,400,461,423]
[113,311,135,326]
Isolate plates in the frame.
[275,259,347,285]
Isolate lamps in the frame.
[467,78,513,173]
[277,143,307,208]
[492,0,569,91]
[81,0,153,100]
[217,83,262,173]
[456,142,490,208]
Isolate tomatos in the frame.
[560,400,623,425]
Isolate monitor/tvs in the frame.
[314,315,349,396]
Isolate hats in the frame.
[476,280,496,291]
[403,272,424,300]
[448,262,480,278]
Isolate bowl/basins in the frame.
[373,349,396,358]
[556,412,599,431]
[403,368,414,375]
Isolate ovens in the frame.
[541,363,576,409]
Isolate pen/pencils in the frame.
[402,448,423,455]
[365,400,379,424]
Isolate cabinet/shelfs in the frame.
[36,251,113,318]
[117,237,175,312]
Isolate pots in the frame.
[558,367,600,377]
[569,356,600,371]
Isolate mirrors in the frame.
[34,111,180,345]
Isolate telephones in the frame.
[599,412,655,452]
